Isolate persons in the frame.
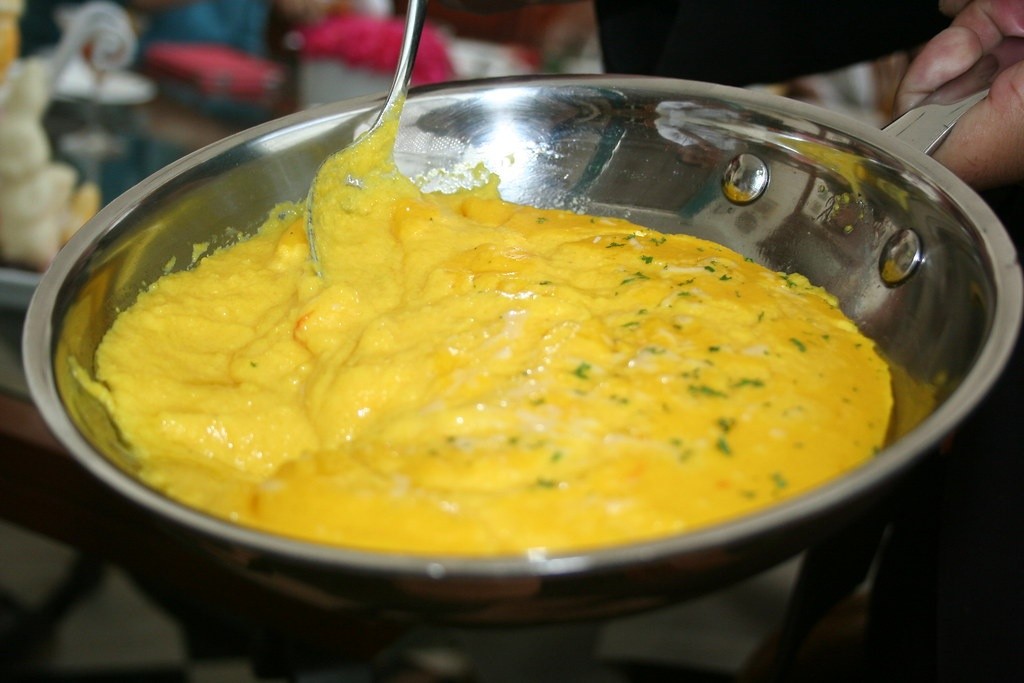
[894,0,1024,196]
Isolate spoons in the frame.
[303,0,427,286]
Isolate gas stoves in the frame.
[1,262,959,683]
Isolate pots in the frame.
[19,34,1024,630]
